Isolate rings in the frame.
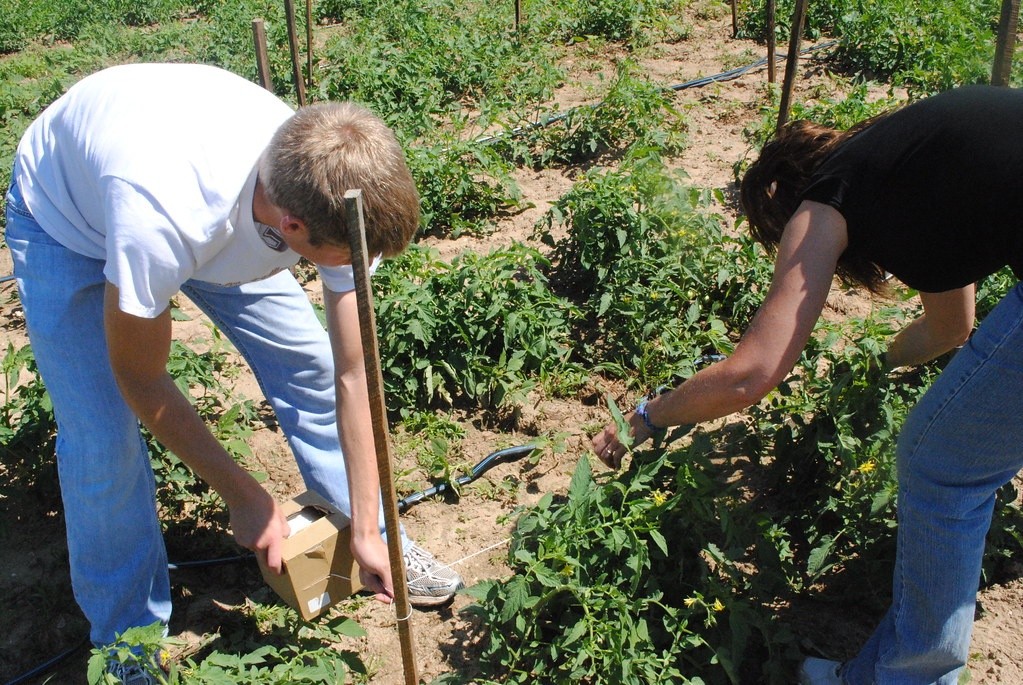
[605,447,613,454]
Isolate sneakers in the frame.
[363,541,465,607]
[103,651,169,685]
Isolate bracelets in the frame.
[635,402,663,433]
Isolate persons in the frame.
[592,86,1023,685]
[5,61,466,685]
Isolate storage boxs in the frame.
[255,490,366,623]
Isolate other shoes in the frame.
[798,657,843,685]
